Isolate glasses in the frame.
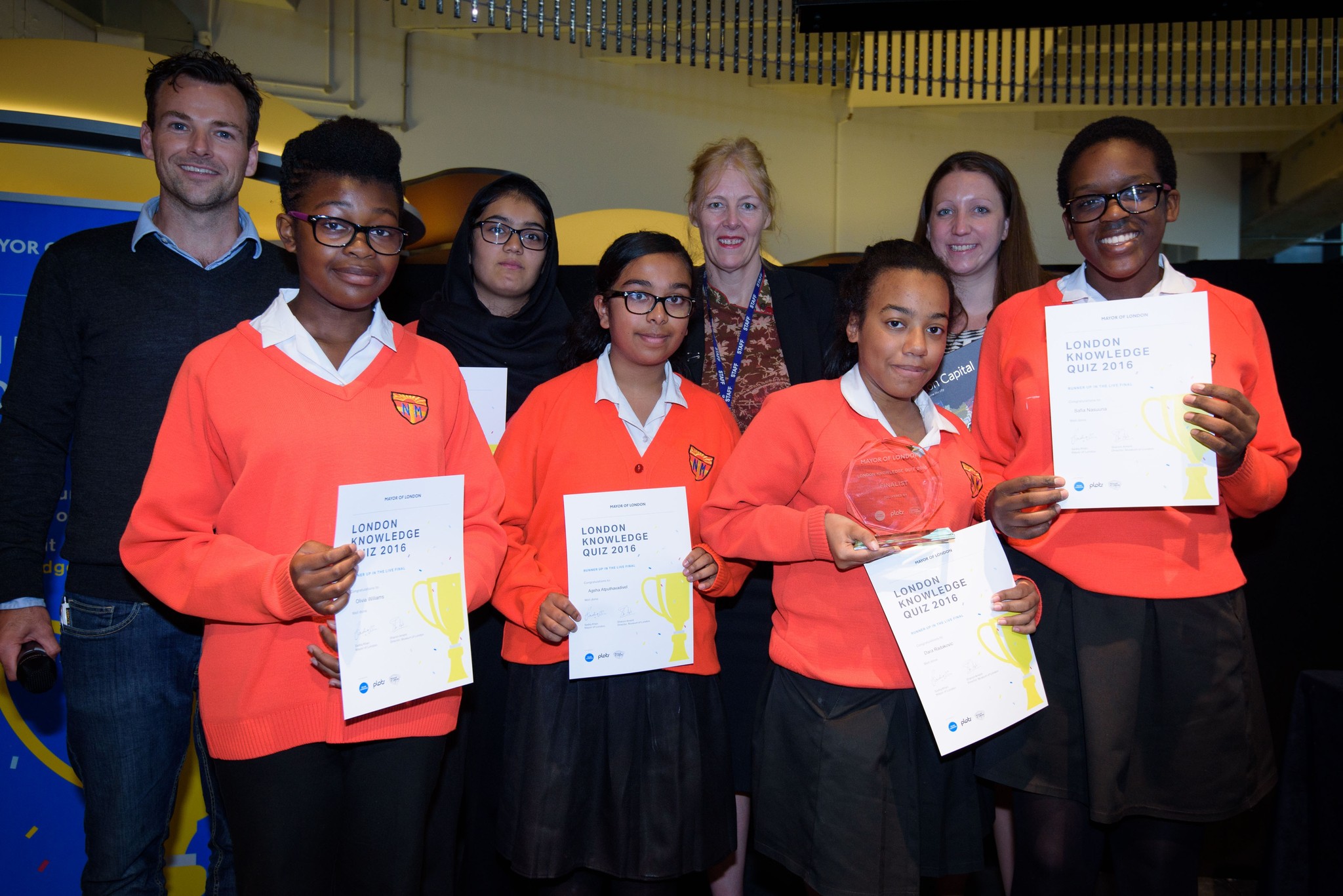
[470,220,553,251]
[1066,183,1173,223]
[604,288,698,318]
[286,207,413,256]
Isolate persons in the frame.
[914,150,1042,896]
[701,240,1042,896]
[119,113,507,896]
[675,138,844,896]
[491,230,759,896]
[972,115,1302,896]
[418,177,591,896]
[0,51,303,896]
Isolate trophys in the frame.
[841,436,955,550]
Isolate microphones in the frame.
[16,640,58,694]
